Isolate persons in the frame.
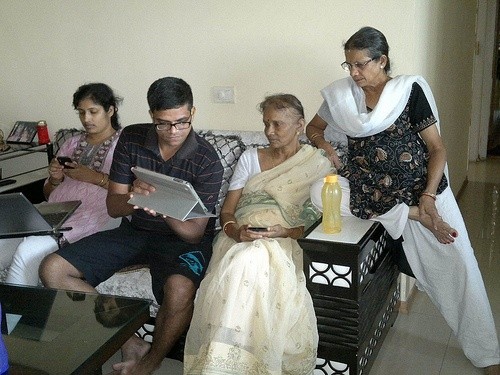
[0,83,124,286]
[183,93,335,375]
[39,77,224,375]
[93,294,144,328]
[306,27,500,374]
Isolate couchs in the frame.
[58,129,349,375]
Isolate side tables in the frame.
[296,219,405,375]
[1,141,55,208]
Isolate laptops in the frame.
[0,192,81,238]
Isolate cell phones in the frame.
[247,227,268,232]
[57,157,74,169]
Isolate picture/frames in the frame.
[7,121,41,144]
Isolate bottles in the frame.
[37,120,49,145]
[321,173,342,234]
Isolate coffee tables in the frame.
[0,284,154,374]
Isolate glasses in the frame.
[152,110,193,130]
[340,56,380,72]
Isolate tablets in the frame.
[132,166,208,215]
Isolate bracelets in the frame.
[310,133,323,141]
[48,177,64,186]
[223,221,236,231]
[98,171,110,187]
[420,192,436,201]
